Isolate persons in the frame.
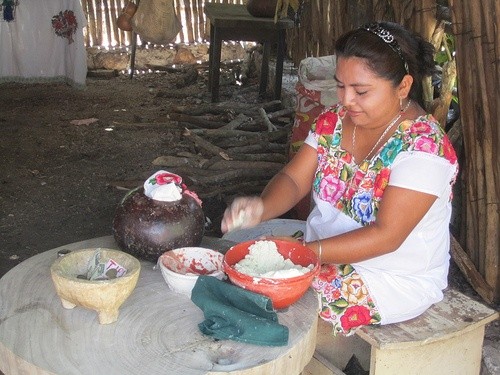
[221,23,460,338]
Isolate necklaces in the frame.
[350,99,412,186]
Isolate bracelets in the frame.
[318,240,322,262]
[296,236,306,248]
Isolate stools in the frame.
[316,287,499,375]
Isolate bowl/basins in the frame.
[222,239,321,310]
[50,248,141,325]
[158,246,226,296]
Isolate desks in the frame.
[204,3,295,103]
[0,230,318,375]
